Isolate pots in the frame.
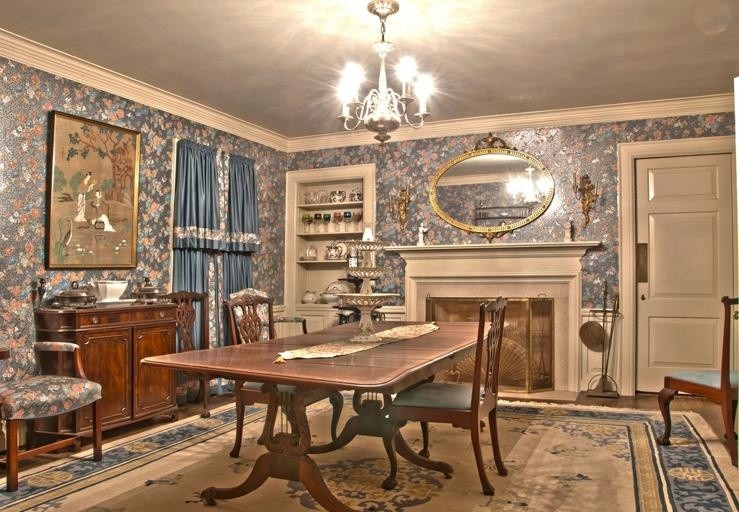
[131,279,168,305]
[52,281,97,309]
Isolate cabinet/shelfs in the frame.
[296,311,339,337]
[34,301,180,451]
[381,313,405,322]
[296,180,364,306]
[475,206,531,226]
[272,312,286,340]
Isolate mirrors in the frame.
[428,133,555,243]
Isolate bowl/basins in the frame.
[93,281,128,303]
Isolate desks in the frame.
[142,320,513,512]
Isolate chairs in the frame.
[230,287,308,345]
[657,296,738,468]
[162,290,217,418]
[381,296,508,497]
[0,298,103,493]
[223,295,344,458]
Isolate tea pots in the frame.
[326,241,343,260]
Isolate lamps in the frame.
[335,0,430,149]
[504,168,550,208]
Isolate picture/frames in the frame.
[46,110,142,272]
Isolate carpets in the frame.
[1,391,739,512]
